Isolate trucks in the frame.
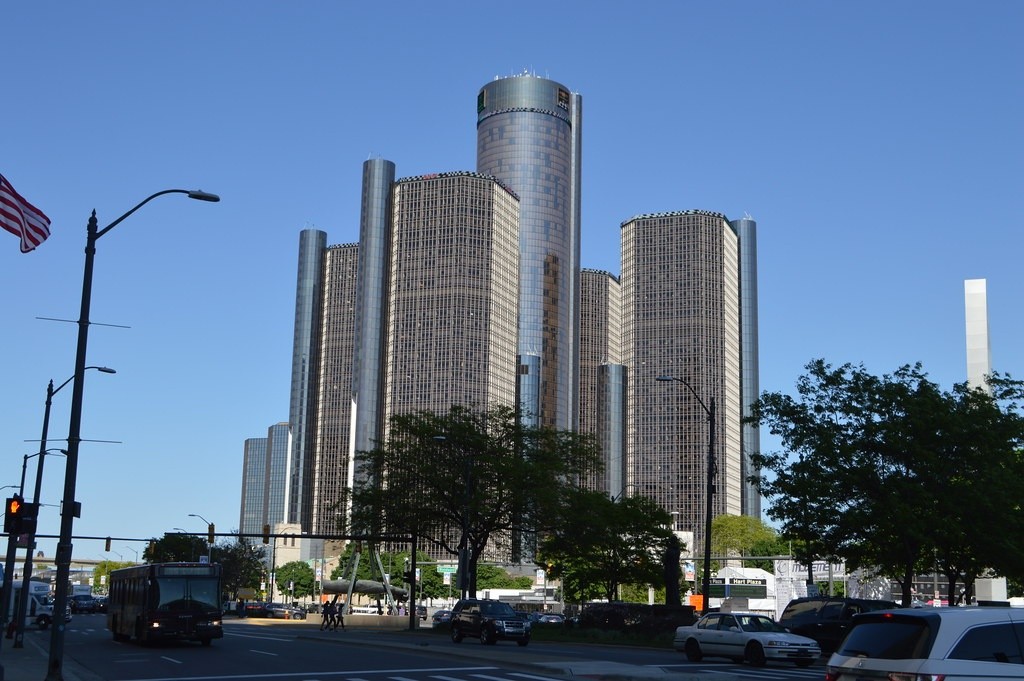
[0,580,72,631]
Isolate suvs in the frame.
[450,597,531,647]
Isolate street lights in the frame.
[14,366,116,648]
[173,528,195,562]
[655,375,715,613]
[270,527,308,602]
[671,511,699,594]
[188,514,211,563]
[46,187,221,681]
[0,448,70,623]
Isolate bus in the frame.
[108,561,224,647]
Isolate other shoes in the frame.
[320,629,324,631]
[329,628,334,631]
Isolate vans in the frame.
[778,596,905,655]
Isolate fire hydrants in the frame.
[284,610,289,620]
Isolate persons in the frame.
[402,604,406,614]
[533,608,540,623]
[320,601,346,632]
[239,599,244,618]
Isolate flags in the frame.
[0,174,51,254]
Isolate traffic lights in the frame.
[8,492,23,517]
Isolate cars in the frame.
[824,605,1024,681]
[431,610,452,630]
[247,602,428,620]
[516,610,567,624]
[64,584,110,621]
[672,612,821,667]
